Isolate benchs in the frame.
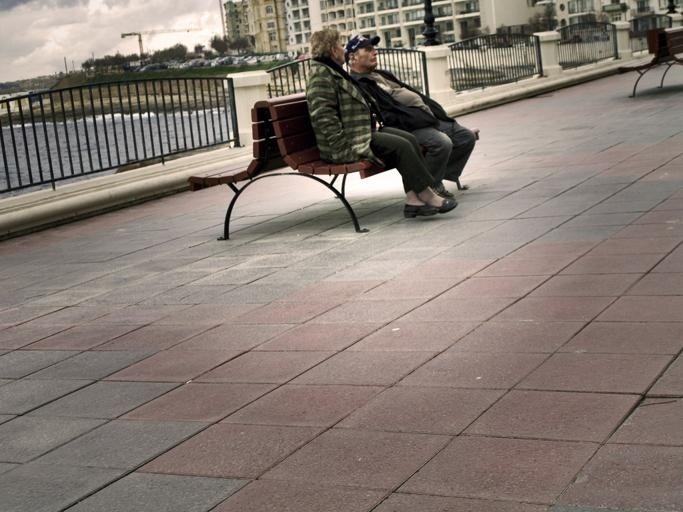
[186,91,481,242]
[614,28,683,97]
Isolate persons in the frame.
[344,34,474,199]
[305,28,458,219]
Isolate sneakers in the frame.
[402,198,459,220]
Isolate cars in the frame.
[124,53,289,72]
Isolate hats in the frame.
[344,34,381,53]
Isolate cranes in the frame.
[121,28,201,60]
